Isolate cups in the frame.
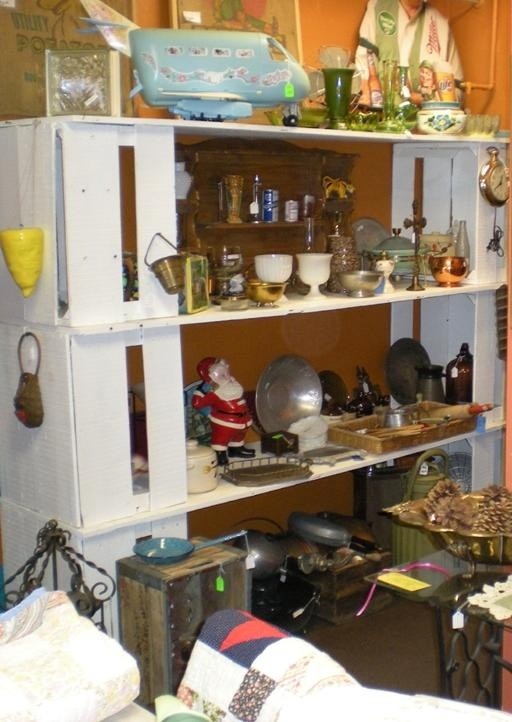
[213,243,293,308]
[223,174,244,224]
[433,68,456,103]
[381,60,404,131]
[428,256,468,288]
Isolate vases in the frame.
[390,64,417,119]
[321,198,363,295]
[320,67,359,132]
[453,215,471,257]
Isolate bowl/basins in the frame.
[250,577,321,636]
[379,496,512,568]
[369,228,418,275]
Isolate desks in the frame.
[360,543,512,709]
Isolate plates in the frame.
[353,218,388,271]
[254,354,324,436]
[317,369,349,415]
[385,337,430,404]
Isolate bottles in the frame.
[233,526,288,580]
[445,219,473,269]
[175,161,193,201]
[415,364,445,401]
[326,234,363,295]
[445,343,473,400]
[186,440,219,495]
[415,101,466,135]
[364,52,383,107]
[372,251,396,295]
[419,231,452,274]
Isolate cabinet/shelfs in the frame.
[0,114,512,644]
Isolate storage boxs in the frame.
[307,536,394,622]
[115,527,250,705]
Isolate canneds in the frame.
[261,189,279,223]
[285,200,299,222]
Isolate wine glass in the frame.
[322,67,354,131]
[296,254,334,299]
[337,270,380,299]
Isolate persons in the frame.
[348,0,464,106]
[193,358,258,463]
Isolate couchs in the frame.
[0,585,510,719]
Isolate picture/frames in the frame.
[169,0,304,74]
[41,49,122,122]
[185,254,211,314]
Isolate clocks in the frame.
[477,143,512,260]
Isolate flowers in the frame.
[315,169,358,237]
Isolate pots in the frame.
[132,525,248,565]
